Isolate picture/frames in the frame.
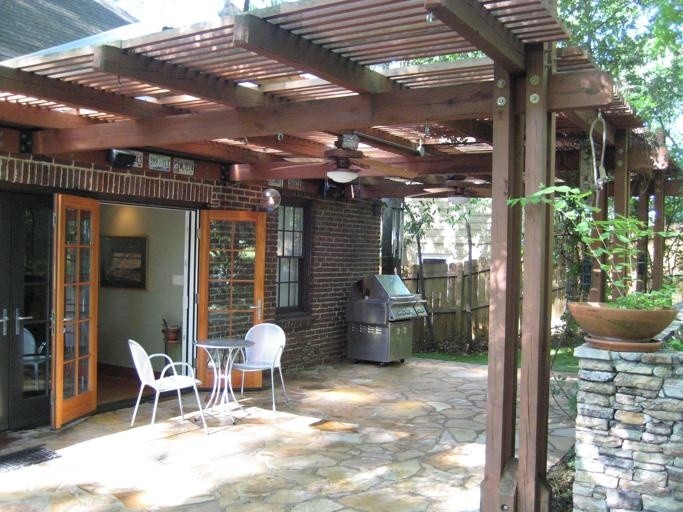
[100,232,149,290]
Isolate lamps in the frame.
[253,188,281,212]
[108,150,136,169]
[325,142,363,184]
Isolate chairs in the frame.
[23,328,51,391]
[233,323,288,412]
[128,339,209,434]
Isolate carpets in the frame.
[0,443,61,473]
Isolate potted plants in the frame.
[505,183,683,352]
